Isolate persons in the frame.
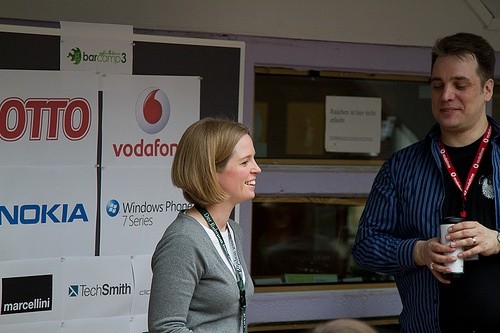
[148,119,261,333]
[353,32,500,333]
[313,318,379,333]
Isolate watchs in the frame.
[497,231,500,243]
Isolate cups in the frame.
[440,217,463,274]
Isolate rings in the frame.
[430,262,434,270]
[472,237,476,245]
